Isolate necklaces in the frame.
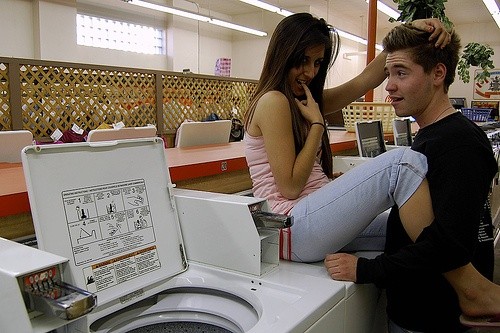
[433,106,453,123]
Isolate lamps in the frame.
[121,0,405,51]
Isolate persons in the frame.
[243,12,500,327]
[325,23,496,333]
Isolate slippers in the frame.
[459,314,500,329]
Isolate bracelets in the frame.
[310,122,325,129]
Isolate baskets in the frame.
[341,99,400,136]
[460,106,492,123]
